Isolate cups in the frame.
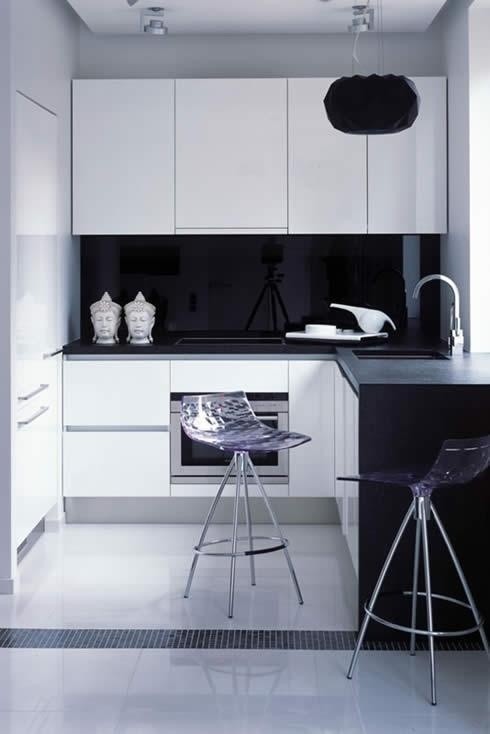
[306,324,354,337]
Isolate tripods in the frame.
[243,264,289,331]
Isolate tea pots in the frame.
[329,302,397,334]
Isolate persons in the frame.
[124,291,157,345]
[89,292,123,344]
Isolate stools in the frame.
[336,439,490,706]
[177,389,310,619]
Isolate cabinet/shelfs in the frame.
[286,74,449,237]
[337,363,485,642]
[2,90,65,594]
[72,76,287,236]
[62,354,335,520]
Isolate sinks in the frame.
[357,355,449,359]
[352,349,443,356]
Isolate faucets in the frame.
[410,270,467,354]
